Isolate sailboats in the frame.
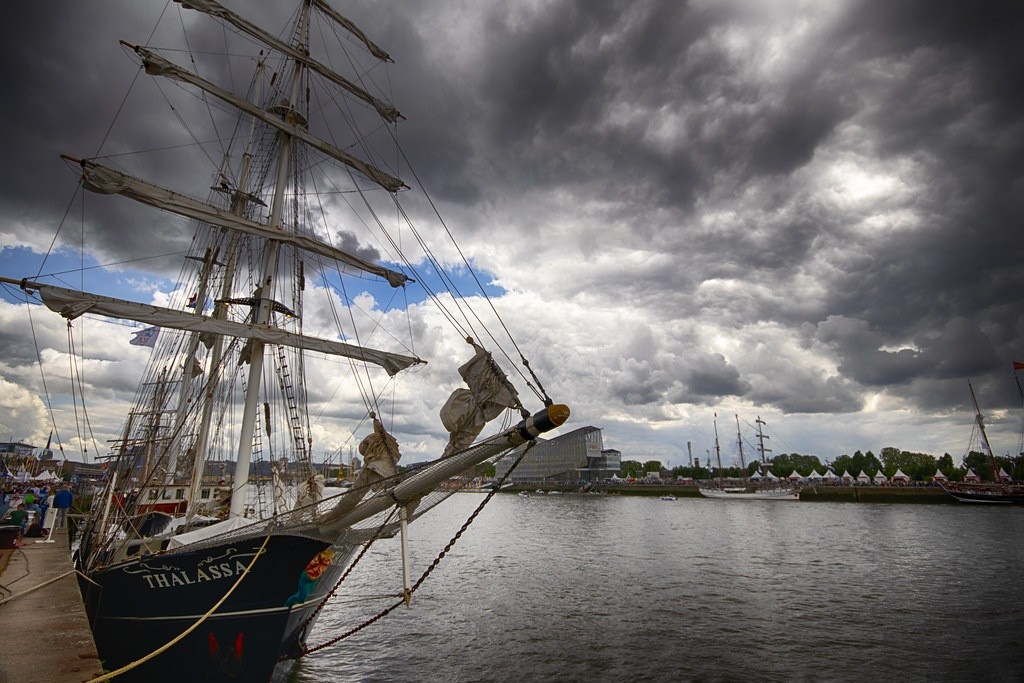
[935,376,1024,507]
[0,0,570,682]
[697,412,804,499]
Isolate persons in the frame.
[111,488,138,524]
[3,481,74,538]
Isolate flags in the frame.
[1013,361,1024,370]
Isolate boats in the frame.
[536,488,544,494]
[518,490,530,498]
[547,490,563,496]
[659,494,678,500]
